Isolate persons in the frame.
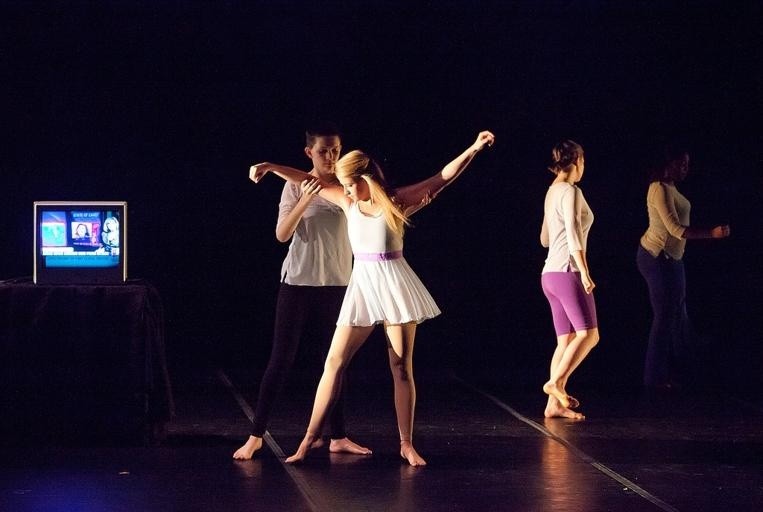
[637,142,730,401]
[540,138,600,421]
[101,217,119,256]
[75,224,90,237]
[232,123,435,459]
[249,130,494,466]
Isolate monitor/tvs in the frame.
[34,201,128,285]
